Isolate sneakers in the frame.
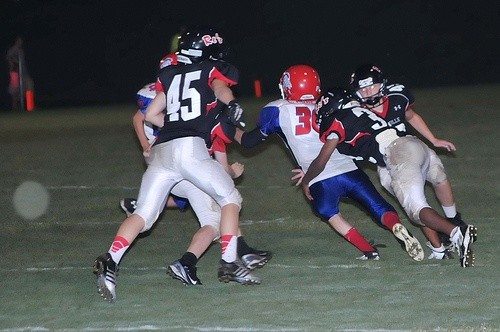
[392,223,425,261]
[120,198,136,217]
[356,247,382,261]
[167,259,202,286]
[240,247,272,271]
[217,259,262,285]
[425,240,455,260]
[449,225,477,267]
[92,252,119,303]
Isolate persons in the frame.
[92,29,273,303]
[348,65,456,259]
[235,65,424,261]
[290,88,478,268]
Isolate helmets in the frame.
[160,25,226,69]
[350,64,389,109]
[316,90,361,123]
[278,65,321,101]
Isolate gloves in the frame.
[225,99,246,129]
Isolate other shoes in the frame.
[447,211,466,226]
